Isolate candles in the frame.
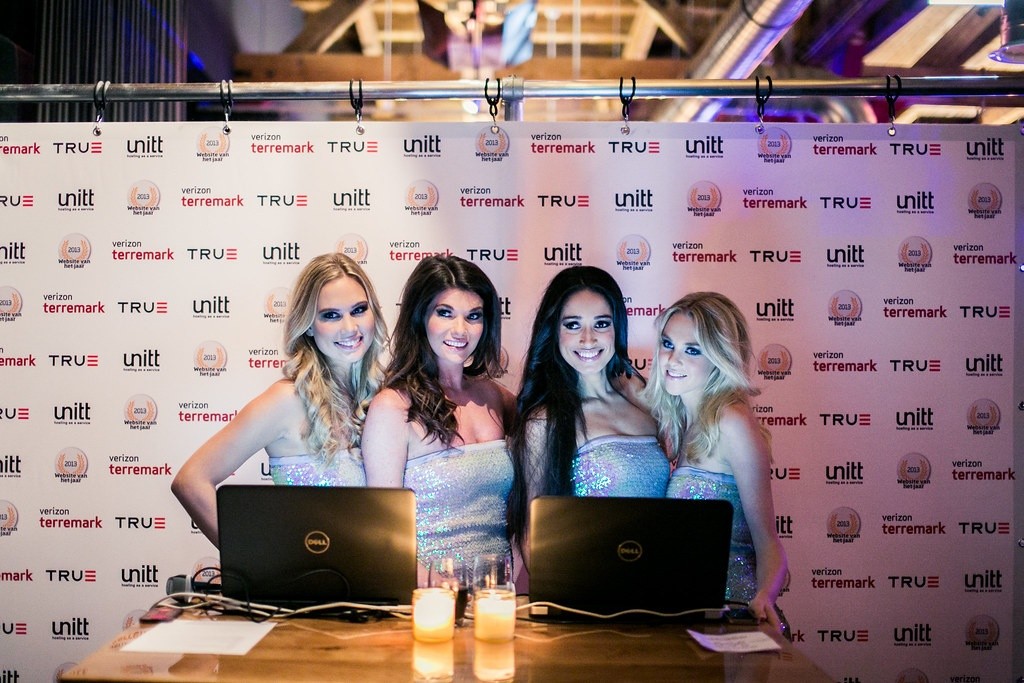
[474,589,516,643]
[414,588,455,643]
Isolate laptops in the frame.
[528,494,734,623]
[216,484,419,615]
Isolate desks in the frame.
[60,609,837,683]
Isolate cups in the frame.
[472,556,512,589]
[409,585,457,643]
[471,582,516,642]
[429,559,469,628]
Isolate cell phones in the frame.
[140,601,184,624]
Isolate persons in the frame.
[171,252,393,552]
[521,265,670,579]
[636,293,796,643]
[361,256,521,597]
